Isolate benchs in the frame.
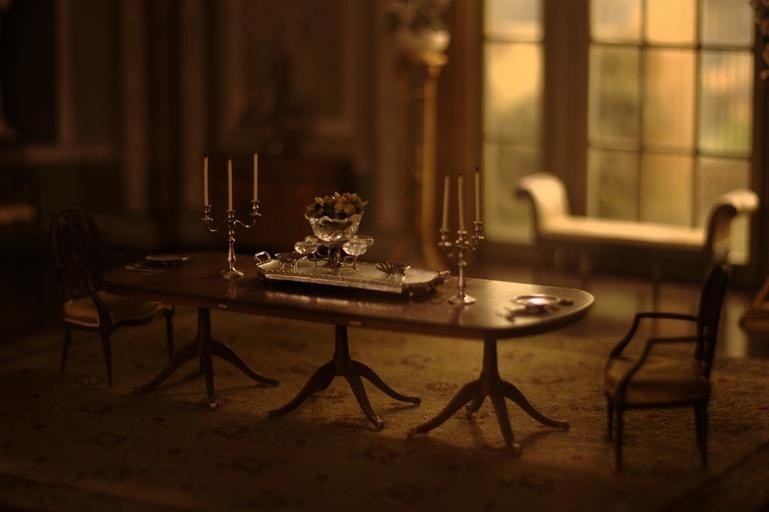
[510,166,763,304]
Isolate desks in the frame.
[97,246,596,457]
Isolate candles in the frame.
[250,148,260,204]
[225,153,235,212]
[455,171,467,232]
[202,152,210,208]
[472,164,482,222]
[440,173,453,229]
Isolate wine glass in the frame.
[345,236,374,271]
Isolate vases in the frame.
[301,210,365,244]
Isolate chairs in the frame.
[602,261,734,473]
[46,203,179,389]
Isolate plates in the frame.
[510,295,559,310]
[145,253,188,263]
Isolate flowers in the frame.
[306,190,370,231]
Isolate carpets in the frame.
[1,300,769,512]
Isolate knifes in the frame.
[126,263,169,274]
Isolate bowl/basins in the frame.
[376,262,410,280]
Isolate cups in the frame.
[294,235,324,261]
